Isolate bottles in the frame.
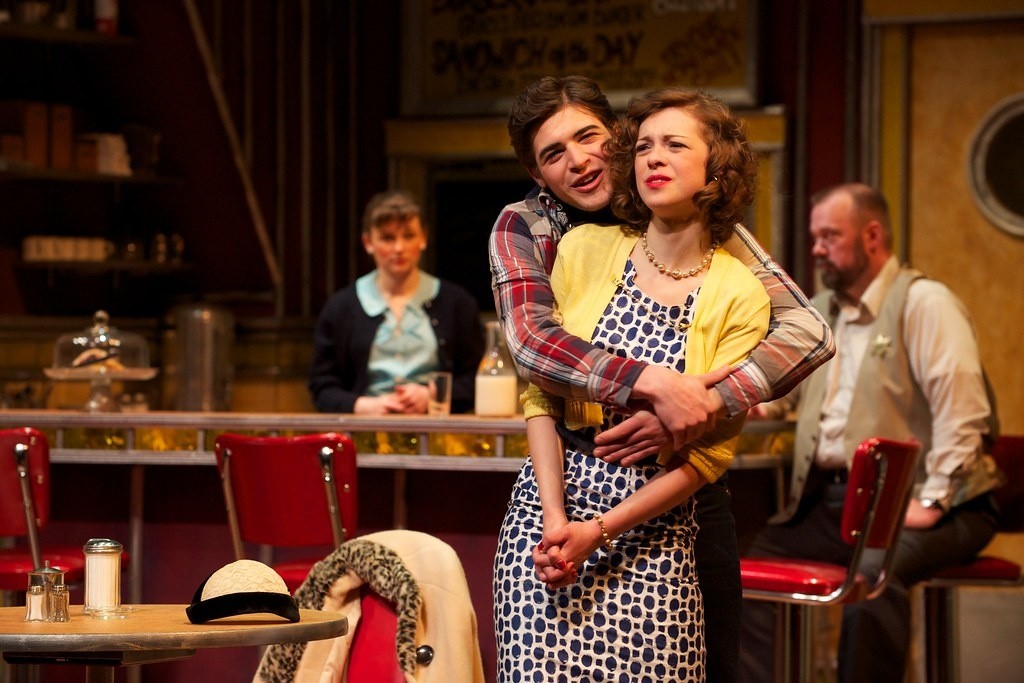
[74,364,127,450]
[83,538,123,614]
[475,322,517,418]
[24,585,48,621]
[49,583,70,622]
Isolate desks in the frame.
[0,604,350,683]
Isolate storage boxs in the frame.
[49,107,73,170]
[0,102,50,170]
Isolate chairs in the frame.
[252,529,486,683]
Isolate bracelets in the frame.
[595,514,613,551]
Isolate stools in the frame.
[0,427,130,683]
[921,434,1024,683]
[740,436,924,683]
[213,431,358,601]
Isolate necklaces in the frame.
[641,230,718,280]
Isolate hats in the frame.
[186,558,302,624]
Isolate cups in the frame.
[427,372,452,417]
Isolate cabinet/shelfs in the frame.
[0,24,194,313]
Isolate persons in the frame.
[311,197,488,417]
[489,75,1005,683]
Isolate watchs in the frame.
[921,499,947,515]
